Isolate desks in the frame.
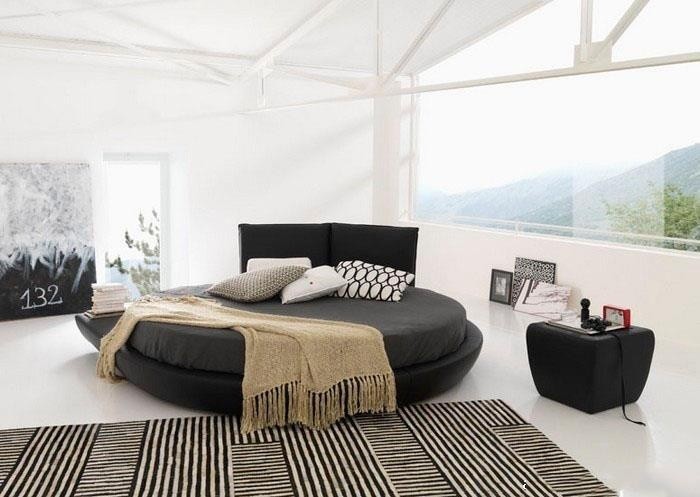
[526,314,655,415]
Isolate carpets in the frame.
[0,399,620,496]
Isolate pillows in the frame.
[205,265,309,304]
[247,257,311,269]
[279,265,349,302]
[312,260,416,303]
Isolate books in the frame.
[83,282,130,320]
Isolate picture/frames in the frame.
[488,269,513,304]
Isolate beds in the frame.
[74,222,483,434]
